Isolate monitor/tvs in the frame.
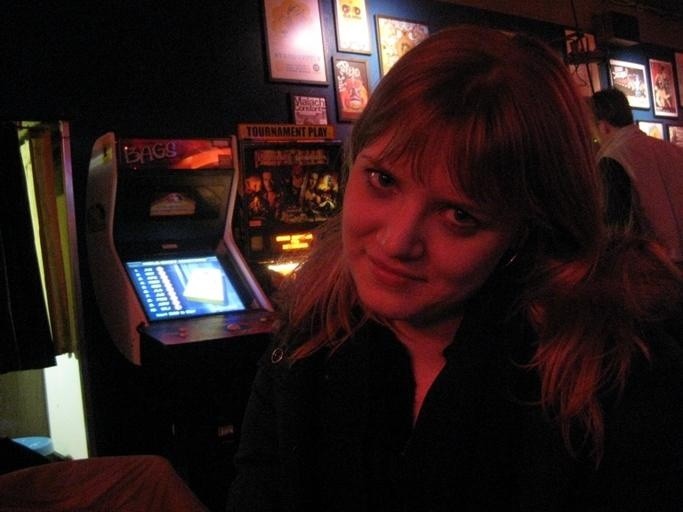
[261,259,305,289]
[123,253,248,324]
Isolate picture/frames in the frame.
[374,13,431,80]
[286,88,329,126]
[605,52,682,146]
[332,57,369,123]
[260,0,329,88]
[331,0,374,54]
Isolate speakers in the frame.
[598,13,640,46]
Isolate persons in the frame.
[586,88,682,264]
[223,25,681,510]
